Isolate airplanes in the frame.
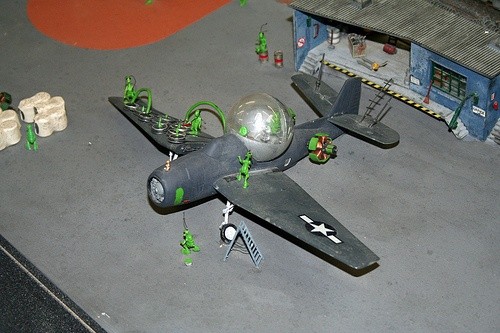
[107,73,399,270]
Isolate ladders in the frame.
[223,221,265,268]
